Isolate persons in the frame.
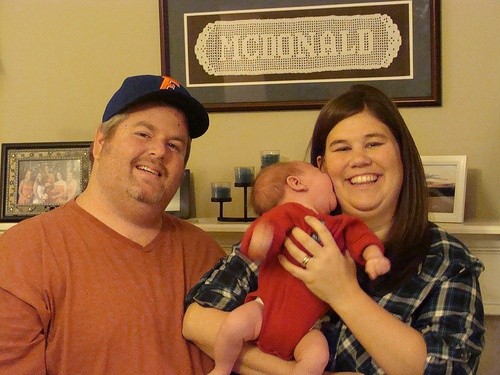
[18,166,76,204]
[206,160,391,375]
[182,85,487,375]
[1,75,236,375]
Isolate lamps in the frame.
[212,149,280,201]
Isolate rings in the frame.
[300,256,310,268]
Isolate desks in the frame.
[194,218,500,316]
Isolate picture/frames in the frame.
[158,0,442,113]
[0,140,94,223]
[420,154,468,224]
[163,168,191,219]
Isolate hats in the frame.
[102,76,209,139]
